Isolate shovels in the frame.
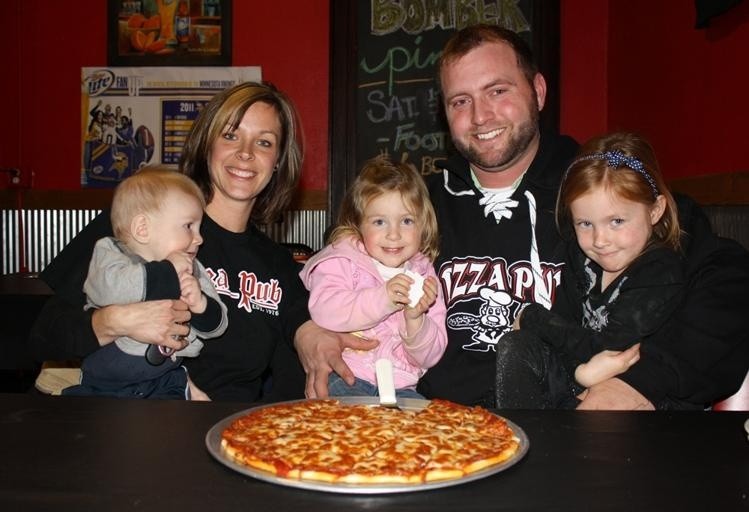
[375,359,402,411]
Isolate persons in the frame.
[297,153,448,401]
[25,82,308,403]
[85,96,134,145]
[496,132,696,410]
[277,23,749,411]
[59,166,230,401]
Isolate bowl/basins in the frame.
[125,28,160,48]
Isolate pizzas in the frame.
[219,399,520,484]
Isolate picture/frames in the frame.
[104,0,234,68]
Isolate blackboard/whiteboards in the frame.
[330,0,562,243]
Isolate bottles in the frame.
[175,1,189,41]
